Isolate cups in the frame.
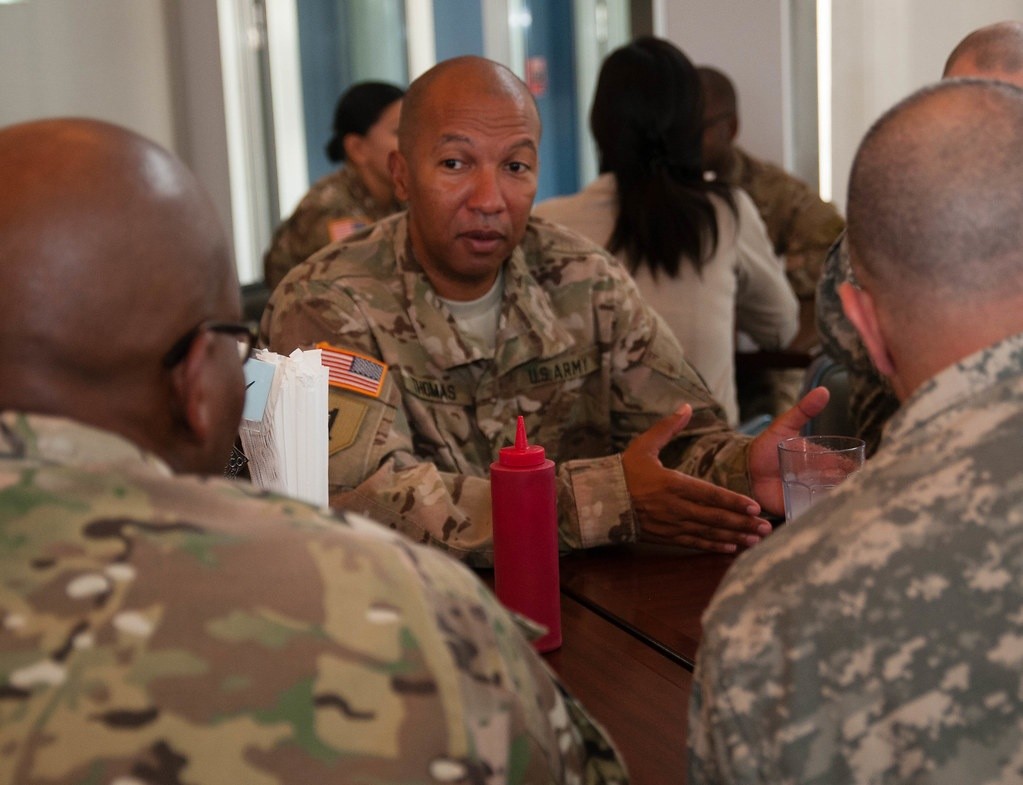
[775,432,864,523]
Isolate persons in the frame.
[686,77,1023,784]
[798,22,1022,466]
[529,35,801,434]
[255,56,866,564]
[265,82,408,294]
[2,118,631,785]
[692,67,846,354]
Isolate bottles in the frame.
[488,411,564,653]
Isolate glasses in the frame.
[159,318,255,370]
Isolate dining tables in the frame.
[558,549,741,676]
[471,571,698,784]
